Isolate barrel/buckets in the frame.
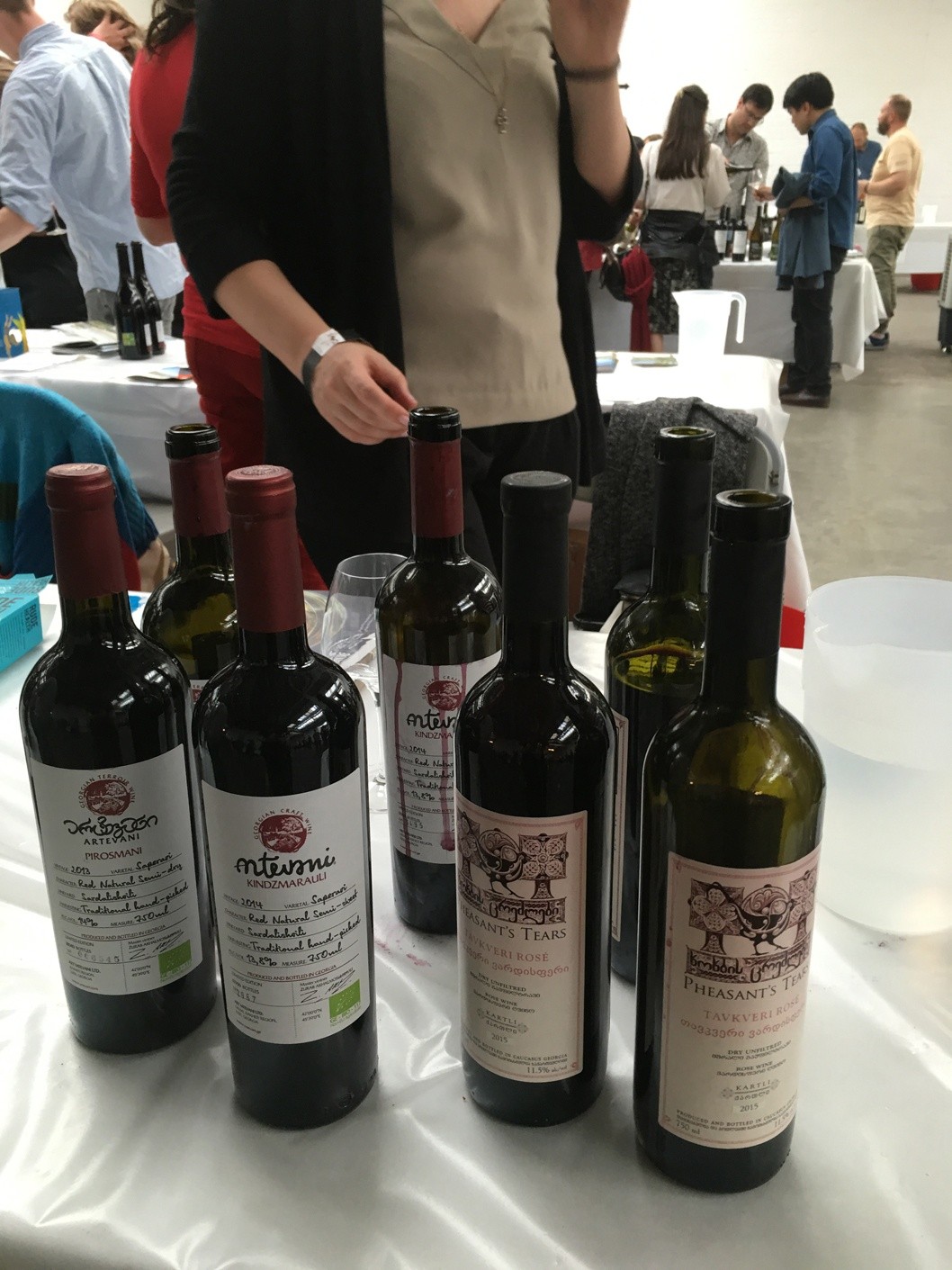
[911,274,942,292]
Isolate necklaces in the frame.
[449,1,516,138]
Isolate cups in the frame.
[809,577,952,933]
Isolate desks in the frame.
[0,314,813,652]
[594,252,888,381]
[848,224,952,348]
[0,579,949,1269]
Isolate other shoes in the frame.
[862,332,890,350]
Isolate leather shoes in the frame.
[778,386,830,408]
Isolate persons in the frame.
[751,71,858,409]
[166,1,648,594]
[132,0,324,597]
[634,85,731,351]
[850,122,883,180]
[631,130,662,154]
[0,0,201,338]
[704,83,773,242]
[856,94,923,350]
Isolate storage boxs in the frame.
[1,572,50,669]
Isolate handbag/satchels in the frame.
[599,247,650,305]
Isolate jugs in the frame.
[671,291,747,373]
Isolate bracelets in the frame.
[563,54,621,83]
[864,181,870,193]
[300,325,347,396]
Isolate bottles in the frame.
[141,423,240,717]
[623,211,642,246]
[749,206,763,261]
[714,205,726,259]
[724,207,733,257]
[771,214,783,260]
[603,425,717,984]
[858,198,866,223]
[763,202,771,241]
[454,471,619,1125]
[191,465,379,1131]
[115,242,153,360]
[633,490,827,1196]
[374,406,502,934]
[18,462,217,1054]
[130,241,166,355]
[732,205,748,262]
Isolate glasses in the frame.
[744,103,765,126]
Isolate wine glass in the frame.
[320,554,408,813]
[748,170,767,206]
